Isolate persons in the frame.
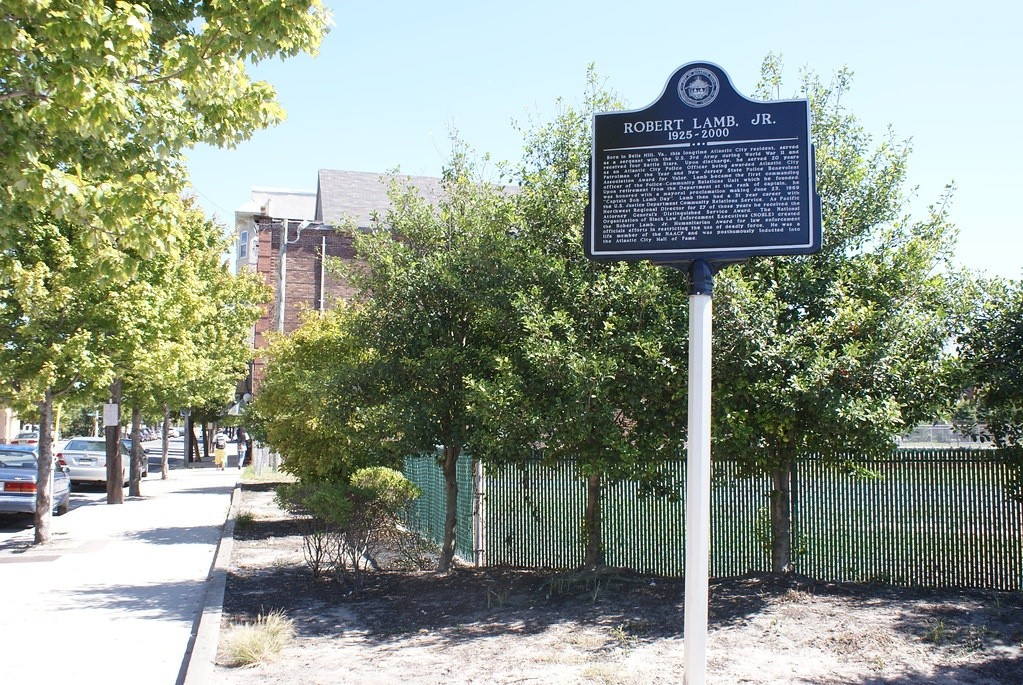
[234,427,249,469]
[212,428,231,470]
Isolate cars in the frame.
[56,437,130,492]
[0,443,71,525]
[140,427,184,442]
[119,438,150,480]
[12,432,39,444]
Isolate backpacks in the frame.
[216,433,225,449]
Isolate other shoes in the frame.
[238,465,241,470]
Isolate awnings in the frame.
[228,399,244,416]
